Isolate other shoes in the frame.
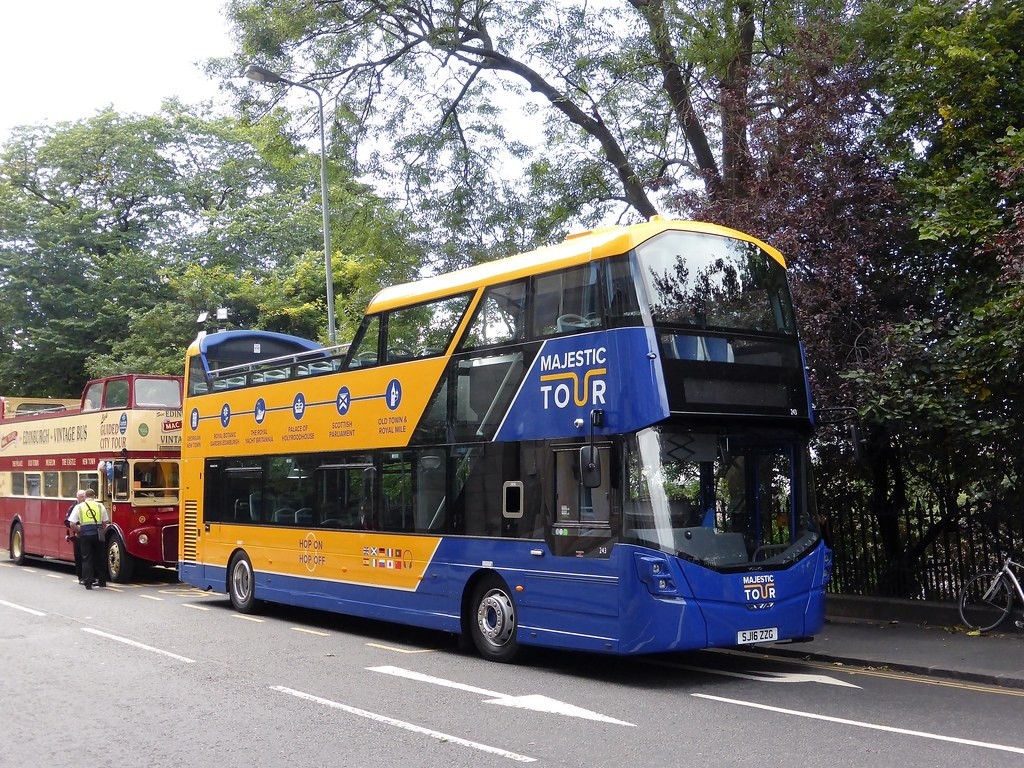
[92,580,106,587]
[86,585,92,589]
[79,579,86,584]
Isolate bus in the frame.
[0,373,181,584]
[175,214,864,667]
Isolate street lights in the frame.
[244,64,336,341]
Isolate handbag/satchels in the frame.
[98,528,105,541]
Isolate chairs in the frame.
[193,346,444,395]
[234,491,349,526]
[557,314,591,334]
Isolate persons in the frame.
[68,488,109,590]
[64,490,97,585]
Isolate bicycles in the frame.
[957,545,1024,634]
